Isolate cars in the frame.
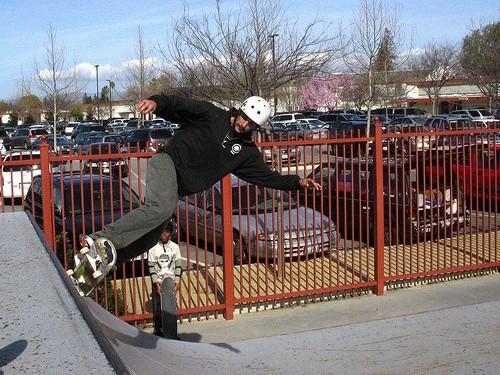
[251,107,500,164]
[425,142,500,202]
[0,117,181,198]
[122,157,342,265]
[25,170,177,271]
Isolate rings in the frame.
[143,102,145,104]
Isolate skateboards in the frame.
[66,236,117,297]
[161,277,177,339]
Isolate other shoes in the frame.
[73,253,81,270]
[78,234,95,250]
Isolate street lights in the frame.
[94,65,100,123]
[105,79,112,118]
[267,34,278,116]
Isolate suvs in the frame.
[287,154,471,248]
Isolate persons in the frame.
[148,221,182,336]
[74,94,324,287]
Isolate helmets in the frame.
[239,96,271,126]
